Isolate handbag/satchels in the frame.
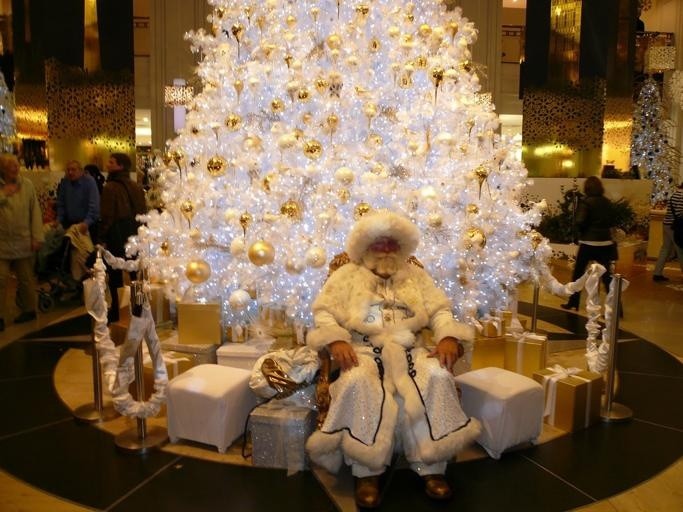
[248,345,321,400]
[670,217,682,248]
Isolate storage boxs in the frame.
[102,272,267,419]
[465,310,603,434]
[249,405,312,474]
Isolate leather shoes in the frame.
[0,318,4,331]
[14,310,38,323]
[652,275,668,281]
[352,476,382,509]
[423,474,452,499]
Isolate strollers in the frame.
[34,225,85,310]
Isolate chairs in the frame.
[315,251,462,430]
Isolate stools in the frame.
[453,367,545,461]
[166,363,252,455]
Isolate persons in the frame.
[650,181,683,282]
[99,152,148,323]
[304,208,484,510]
[84,163,106,195]
[0,152,45,332]
[53,158,102,237]
[558,175,630,320]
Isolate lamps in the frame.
[163,77,195,135]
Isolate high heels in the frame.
[560,300,579,311]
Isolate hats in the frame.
[346,212,423,265]
